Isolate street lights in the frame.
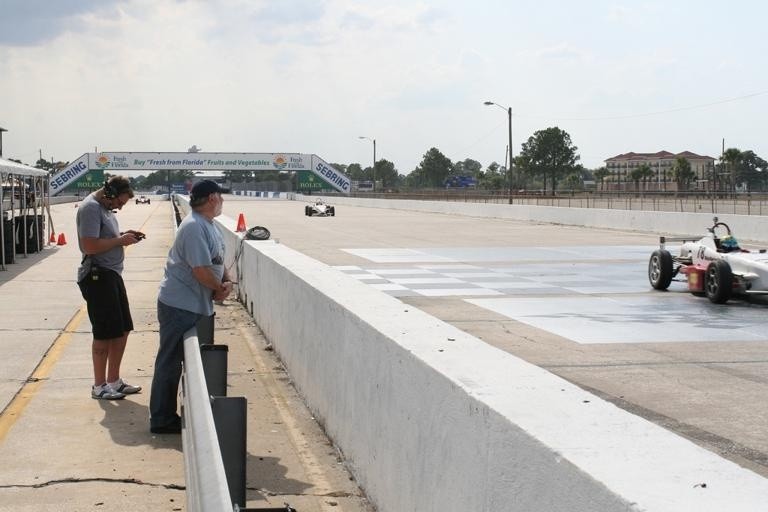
[481,99,515,204]
[357,135,377,194]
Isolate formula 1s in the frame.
[135,194,151,205]
[304,197,336,217]
[646,214,768,303]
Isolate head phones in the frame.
[102,181,119,200]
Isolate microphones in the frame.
[111,209,117,213]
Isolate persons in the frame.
[147,177,238,436]
[73,173,147,401]
[720,235,738,252]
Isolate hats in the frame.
[191,179,230,202]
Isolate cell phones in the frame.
[134,236,144,238]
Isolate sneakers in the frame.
[150,415,182,434]
[91,382,126,400]
[106,378,142,394]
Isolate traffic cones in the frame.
[233,214,248,233]
[49,231,66,246]
